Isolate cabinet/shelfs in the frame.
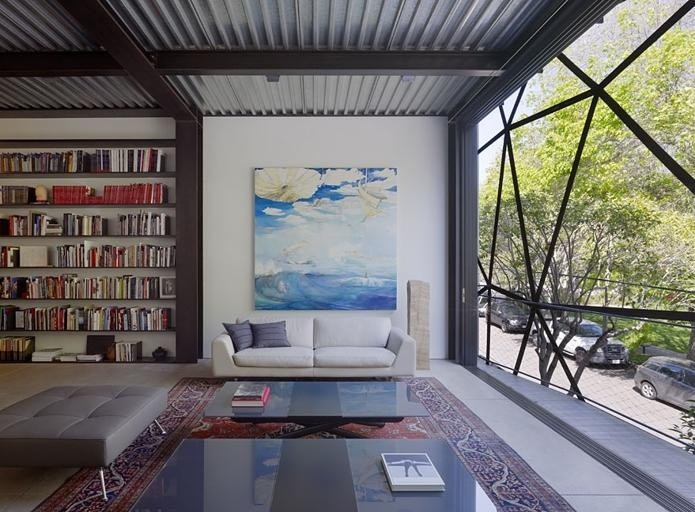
[1,137,179,363]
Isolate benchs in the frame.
[0,383,169,502]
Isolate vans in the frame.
[633,355,695,417]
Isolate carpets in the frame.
[24,376,575,512]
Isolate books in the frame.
[0,144,176,363]
[232,383,270,407]
[381,452,447,491]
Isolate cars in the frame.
[477,278,555,336]
[531,314,630,369]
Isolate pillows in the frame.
[222,318,291,351]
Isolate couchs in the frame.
[210,312,417,383]
